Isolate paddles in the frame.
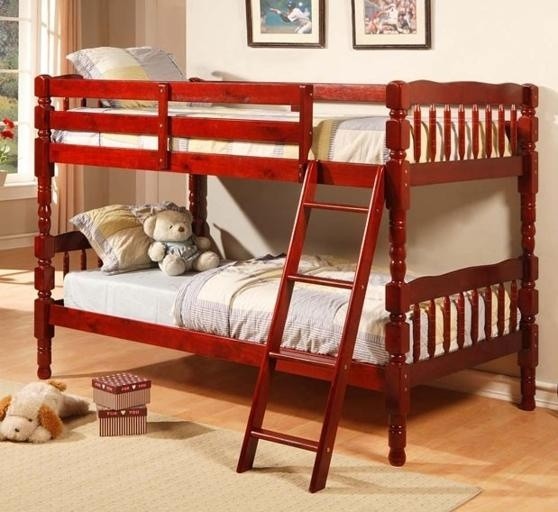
[0,375,483,512]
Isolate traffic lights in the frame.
[64,46,190,108]
[68,203,179,276]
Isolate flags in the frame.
[0,170,8,186]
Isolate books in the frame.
[95,403,148,437]
[91,372,152,411]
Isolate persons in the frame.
[0,379,90,445]
[268,0,310,33]
[363,0,415,34]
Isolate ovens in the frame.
[32,71,540,466]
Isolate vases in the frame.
[0,118,16,168]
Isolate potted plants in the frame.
[245,0,326,49]
[350,0,432,50]
